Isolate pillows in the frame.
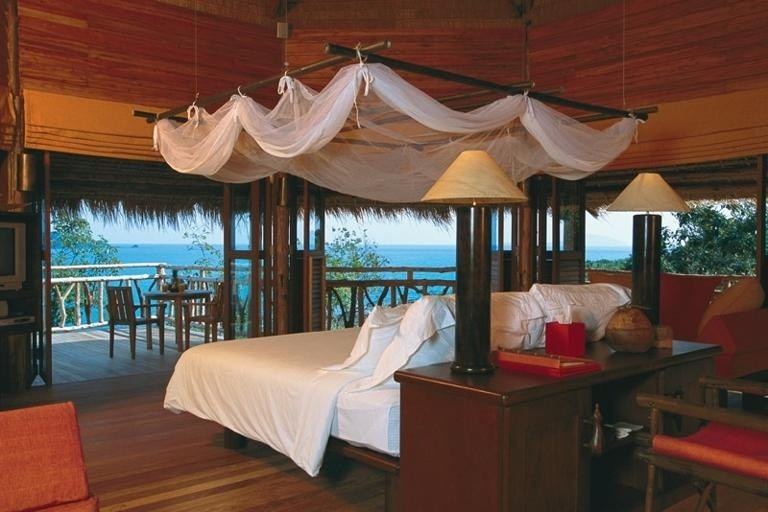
[696,275,765,340]
[530,283,631,349]
[321,303,411,377]
[354,292,546,391]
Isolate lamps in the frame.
[421,149,528,378]
[606,171,692,323]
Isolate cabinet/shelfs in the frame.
[394,339,725,511]
[0,289,37,395]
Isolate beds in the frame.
[184,326,400,474]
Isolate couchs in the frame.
[585,268,768,380]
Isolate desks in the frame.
[143,289,211,353]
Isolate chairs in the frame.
[1,401,100,512]
[634,376,768,512]
[104,285,168,361]
[185,281,236,350]
